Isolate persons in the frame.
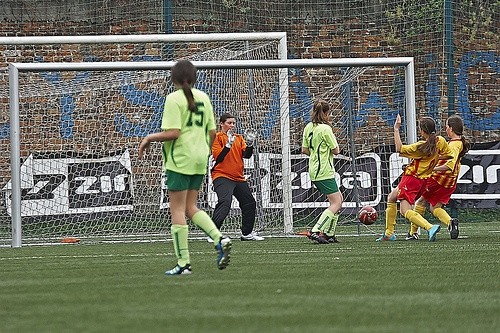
[138,59,232,276]
[376,114,455,243]
[302,99,343,245]
[405,115,470,242]
[207,114,265,244]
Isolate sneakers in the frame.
[405,232,419,241]
[240,232,265,241]
[375,231,397,241]
[427,224,441,242]
[165,264,192,275]
[215,236,232,270]
[448,218,460,240]
[306,229,330,244]
[206,236,215,243]
[323,233,339,243]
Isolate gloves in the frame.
[243,129,257,147]
[225,129,236,148]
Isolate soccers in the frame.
[358,206,377,226]
